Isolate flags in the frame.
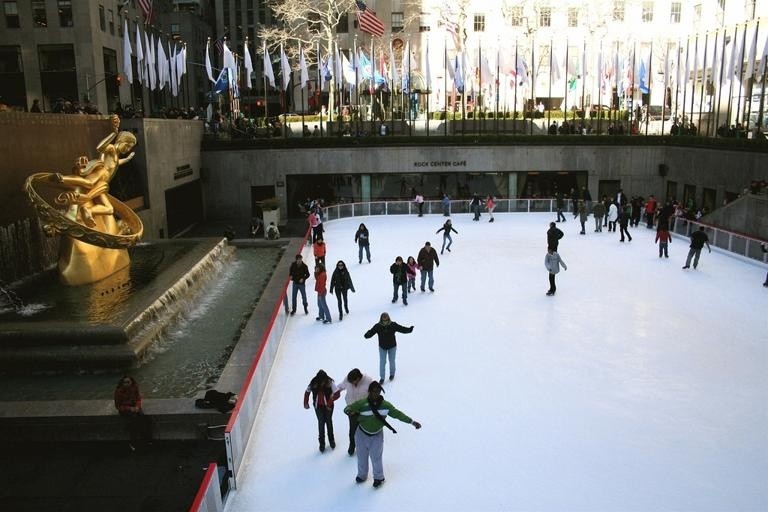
[355,0,384,36]
[138,0,152,25]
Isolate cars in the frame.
[574,103,610,111]
[277,105,361,117]
[752,115,768,134]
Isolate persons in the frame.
[114,375,142,452]
[537,100,768,143]
[47,114,137,227]
[365,312,414,384]
[343,381,421,489]
[303,369,340,451]
[336,368,373,457]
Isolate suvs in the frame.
[647,105,671,121]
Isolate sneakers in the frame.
[356,475,385,487]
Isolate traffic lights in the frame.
[116,77,122,87]
[255,98,264,106]
